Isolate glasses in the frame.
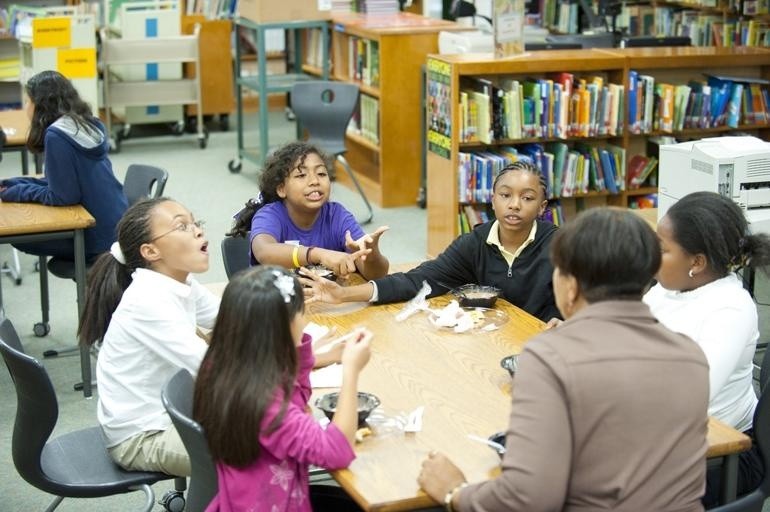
[152,219,202,241]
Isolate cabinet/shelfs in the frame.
[0,27,103,110]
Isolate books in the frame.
[0,0,237,41]
[305,28,378,142]
[629,72,769,207]
[459,77,627,233]
[537,0,770,48]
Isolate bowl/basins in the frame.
[316,392,380,422]
[502,354,534,371]
[297,263,337,283]
[487,430,507,459]
[452,284,501,307]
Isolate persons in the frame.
[0,70,129,261]
[223,143,389,280]
[77,197,217,477]
[642,191,769,435]
[193,263,374,512]
[416,206,712,512]
[299,160,562,330]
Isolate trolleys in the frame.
[94,21,209,152]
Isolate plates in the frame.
[319,408,406,442]
[431,309,510,337]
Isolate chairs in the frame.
[703,377,770,512]
[0,318,179,512]
[43,163,168,361]
[756,341,770,394]
[160,368,219,512]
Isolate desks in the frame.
[0,109,43,175]
[0,173,98,399]
[195,254,753,512]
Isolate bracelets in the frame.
[444,480,472,512]
[292,242,301,269]
[306,243,319,267]
